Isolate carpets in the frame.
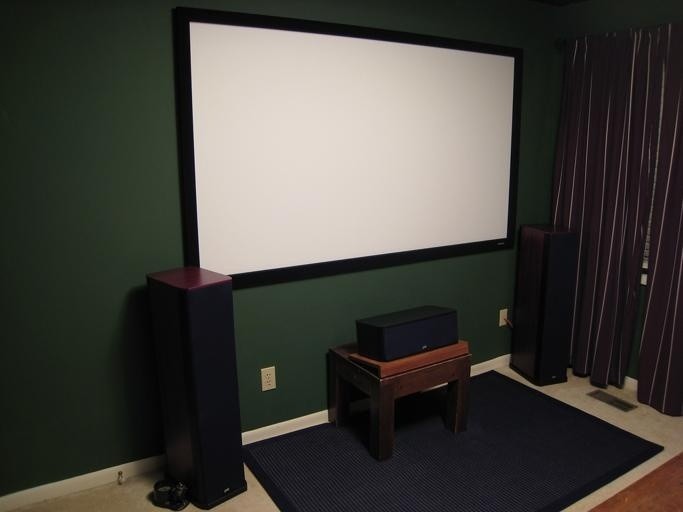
[241,370,665,512]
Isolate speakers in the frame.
[356,305,459,363]
[146,266,248,511]
[509,222,571,387]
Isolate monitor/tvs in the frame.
[171,7,523,290]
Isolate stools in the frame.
[327,345,472,462]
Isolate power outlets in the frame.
[261,367,277,393]
